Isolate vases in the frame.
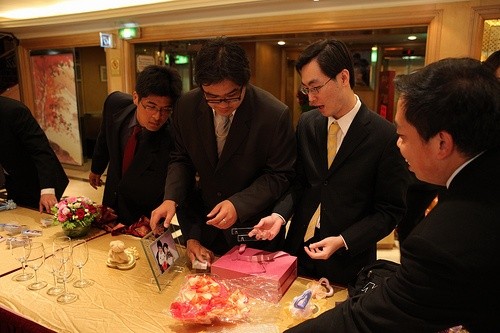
[62,219,91,238]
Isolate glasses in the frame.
[201,86,243,104]
[216,112,232,160]
[140,100,174,114]
[300,77,333,95]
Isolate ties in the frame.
[122,125,139,178]
[304,122,340,242]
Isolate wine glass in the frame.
[55,255,78,303]
[9,234,32,280]
[53,236,76,283]
[43,245,65,295]
[24,242,47,290]
[69,239,94,287]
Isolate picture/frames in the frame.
[140,222,188,292]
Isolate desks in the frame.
[0,203,349,333]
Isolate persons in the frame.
[149,36,297,252]
[155,241,176,273]
[283,49,500,333]
[248,39,409,285]
[0,95,70,214]
[89,65,214,270]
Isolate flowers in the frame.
[48,194,103,231]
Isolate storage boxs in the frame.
[211,245,298,305]
